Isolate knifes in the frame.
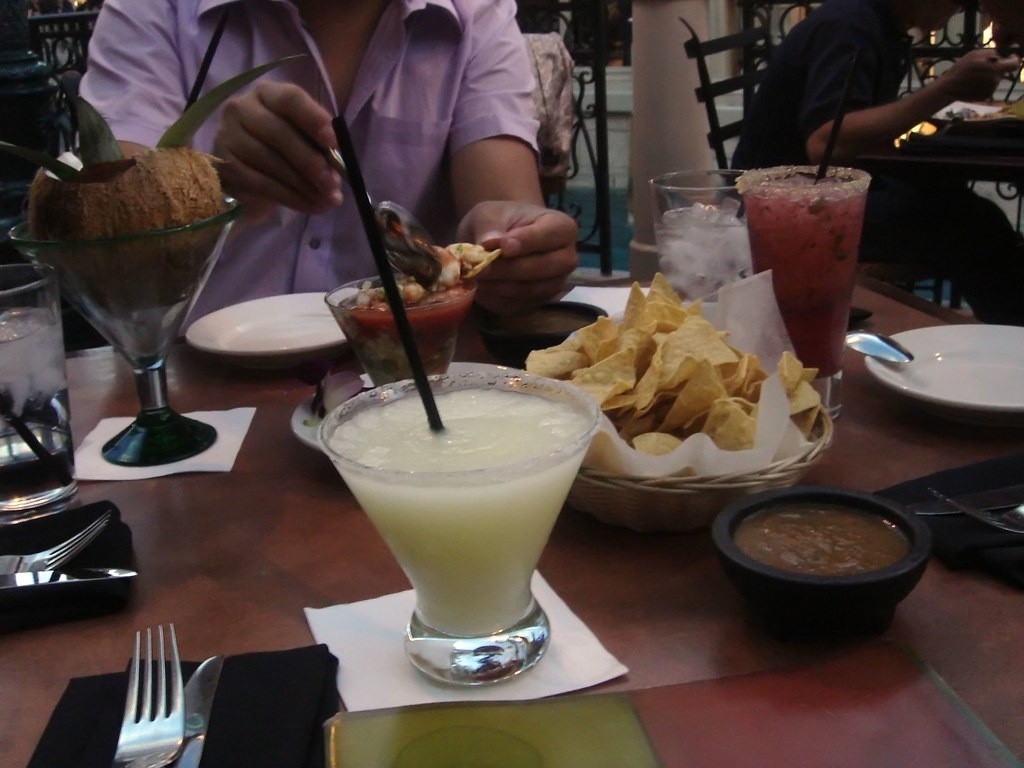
[170,653,225,768]
[905,493,1021,514]
[0,567,138,587]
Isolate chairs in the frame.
[678,15,962,308]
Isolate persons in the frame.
[76,1,579,340]
[729,0,1024,326]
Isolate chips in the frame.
[446,243,501,278]
[524,272,820,455]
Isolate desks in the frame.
[1,273,1024,768]
[854,110,1023,182]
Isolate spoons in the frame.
[317,142,441,287]
[845,328,914,363]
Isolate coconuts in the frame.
[27,150,224,311]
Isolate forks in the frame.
[928,488,1024,534]
[112,622,185,767]
[0,508,111,574]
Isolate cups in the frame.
[318,368,600,686]
[645,167,752,303]
[739,164,870,419]
[324,271,478,389]
[0,262,80,525]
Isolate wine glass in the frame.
[7,193,241,467]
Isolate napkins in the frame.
[25,641,338,768]
[868,446,1023,590]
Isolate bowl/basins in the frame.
[709,482,934,645]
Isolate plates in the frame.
[183,290,347,368]
[861,323,1023,423]
